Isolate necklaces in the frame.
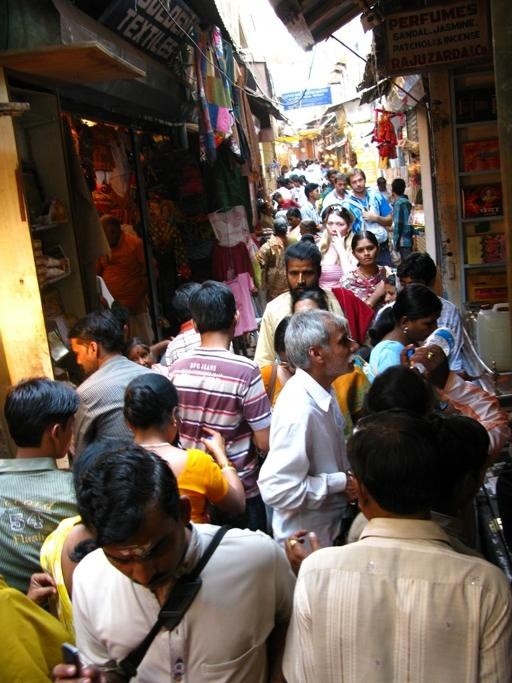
[138,441,170,448]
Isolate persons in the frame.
[384,274,398,304]
[374,175,400,264]
[366,344,512,458]
[292,290,331,317]
[285,208,304,240]
[339,230,399,311]
[67,308,172,470]
[314,203,355,292]
[365,283,443,390]
[161,279,235,371]
[94,215,148,318]
[53,445,298,683]
[346,167,394,267]
[0,376,82,613]
[272,193,301,211]
[343,416,493,550]
[166,280,273,533]
[254,232,376,372]
[40,442,144,648]
[320,170,338,198]
[1,573,73,683]
[321,173,353,208]
[122,373,246,524]
[390,178,414,258]
[256,217,300,304]
[260,316,370,440]
[276,158,328,201]
[278,409,512,683]
[370,250,466,379]
[126,329,172,370]
[300,183,324,224]
[256,308,358,555]
[257,201,275,229]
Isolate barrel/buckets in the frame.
[476,302,512,373]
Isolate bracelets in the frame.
[220,467,237,471]
[218,463,238,468]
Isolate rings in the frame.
[427,351,432,359]
[289,539,297,546]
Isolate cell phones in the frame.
[59,641,84,681]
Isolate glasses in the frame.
[277,358,294,369]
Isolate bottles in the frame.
[408,328,456,374]
